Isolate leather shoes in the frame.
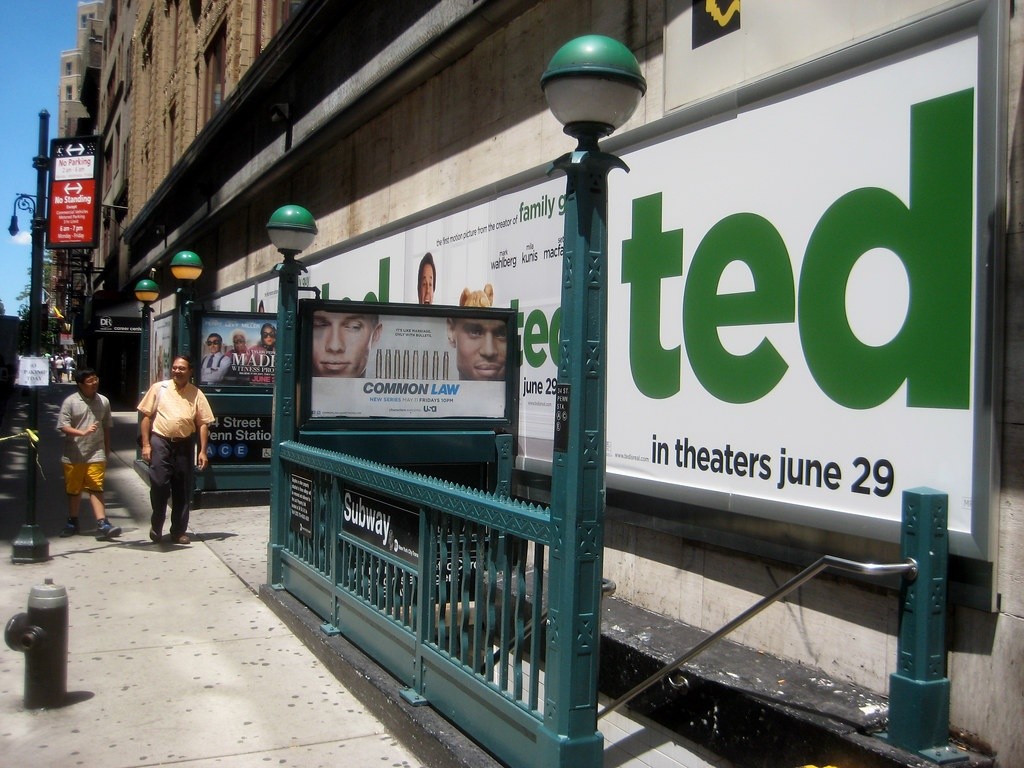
[171,533,191,543]
[149,526,162,543]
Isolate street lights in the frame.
[540,34,648,768]
[264,205,319,584]
[135,280,160,461]
[171,249,205,358]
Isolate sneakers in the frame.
[59,516,80,537]
[96,515,122,541]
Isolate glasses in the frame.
[82,377,99,386]
[263,331,275,338]
[233,338,244,343]
[207,340,219,346]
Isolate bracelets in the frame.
[143,442,151,447]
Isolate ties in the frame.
[206,355,215,368]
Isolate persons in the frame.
[446,317,507,381]
[137,350,215,544]
[312,310,383,378]
[55,353,77,383]
[201,323,276,385]
[56,366,121,541]
[417,251,436,304]
[259,300,264,313]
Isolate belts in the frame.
[152,432,193,443]
